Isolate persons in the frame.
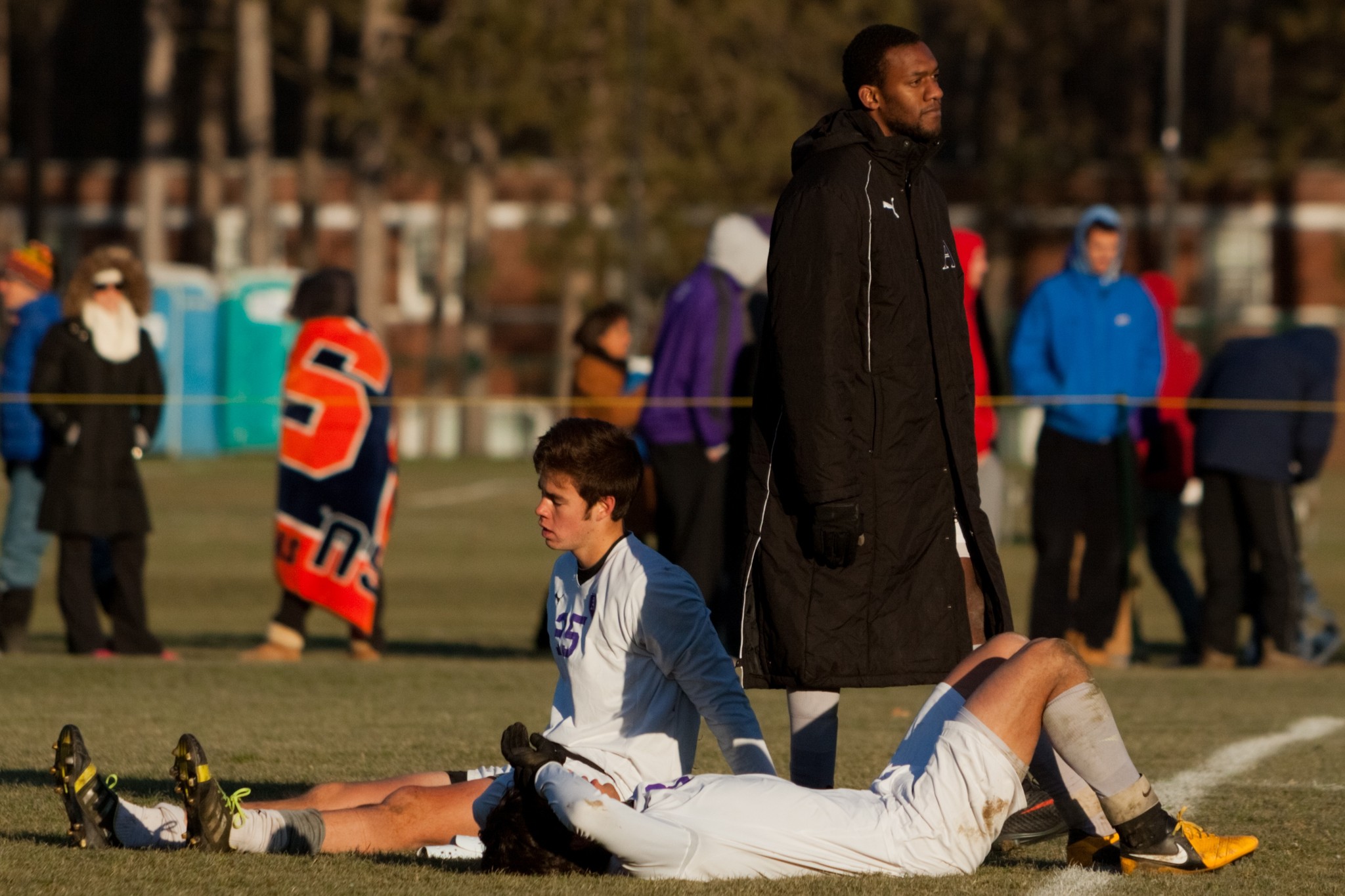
[946,223,1016,647]
[37,243,177,661]
[480,632,1259,882]
[233,265,407,661]
[5,221,114,645]
[643,209,771,665]
[52,417,781,860]
[713,27,1070,855]
[1186,319,1342,659]
[1136,267,1218,669]
[567,297,651,443]
[1008,205,1162,667]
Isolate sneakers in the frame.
[49,724,126,852]
[169,732,239,855]
[1259,636,1320,670]
[1064,827,1139,874]
[1116,813,1259,877]
[238,622,306,668]
[344,637,381,659]
[1199,644,1237,670]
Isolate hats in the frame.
[5,237,54,292]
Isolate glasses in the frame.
[92,278,126,292]
